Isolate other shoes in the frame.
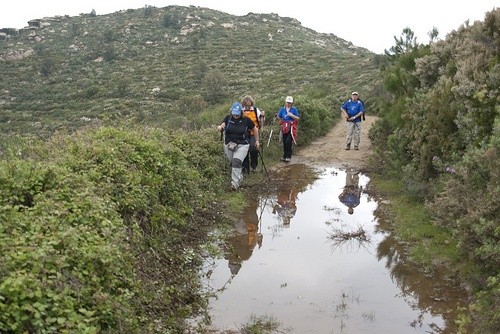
[252,169,257,173]
[243,168,250,177]
[346,144,350,150]
[280,156,291,162]
[231,186,238,194]
[354,146,358,150]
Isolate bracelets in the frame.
[353,117,355,119]
[256,141,259,143]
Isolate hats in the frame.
[286,96,294,103]
[231,102,242,115]
[352,92,358,95]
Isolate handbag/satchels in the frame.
[227,141,238,152]
[254,107,265,129]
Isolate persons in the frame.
[339,168,363,214]
[218,102,259,191]
[242,96,265,174]
[241,208,264,261]
[275,96,299,162]
[272,190,297,228]
[340,92,364,150]
